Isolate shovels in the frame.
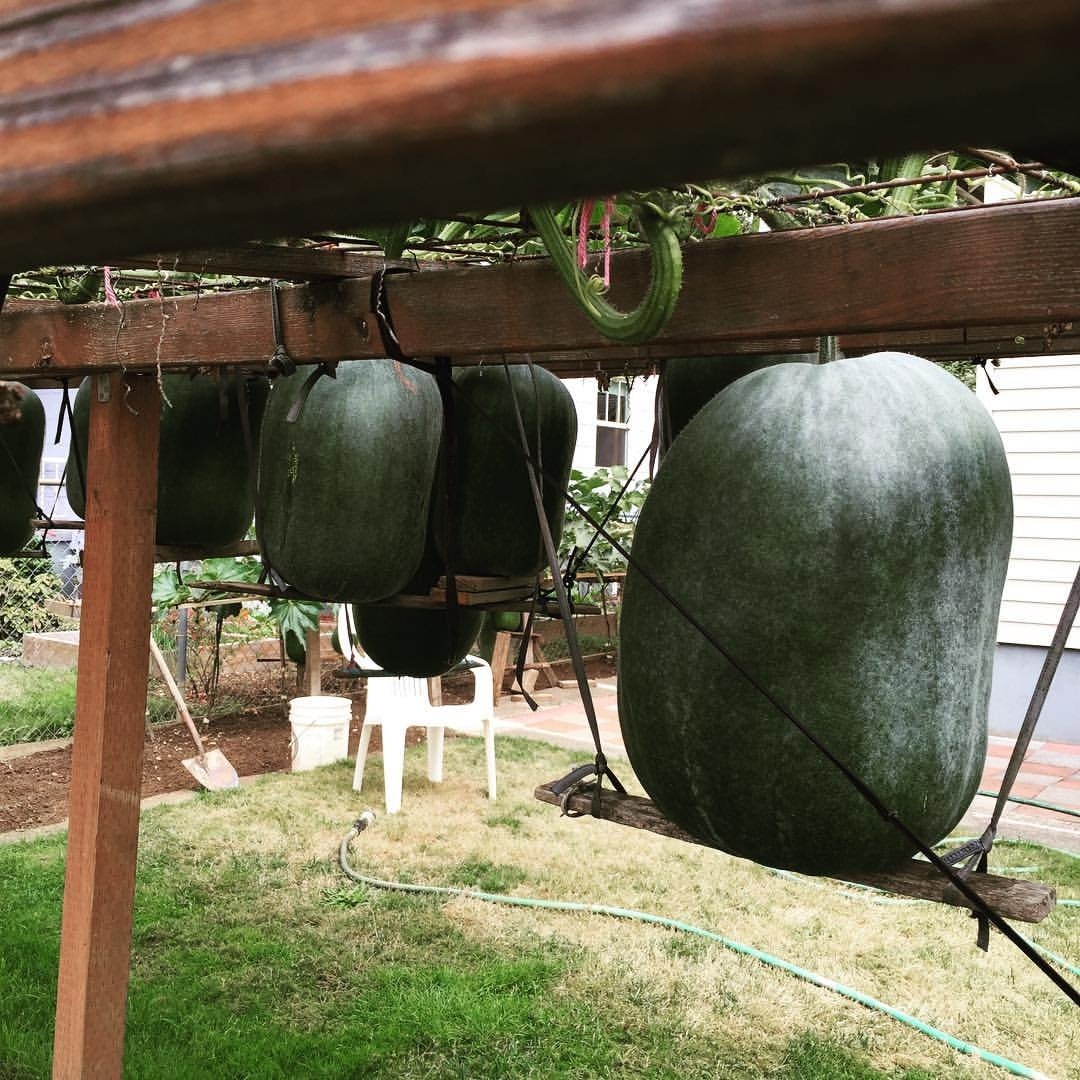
[150,635,239,792]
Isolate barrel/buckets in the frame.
[286,695,353,772]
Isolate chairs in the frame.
[338,604,497,812]
